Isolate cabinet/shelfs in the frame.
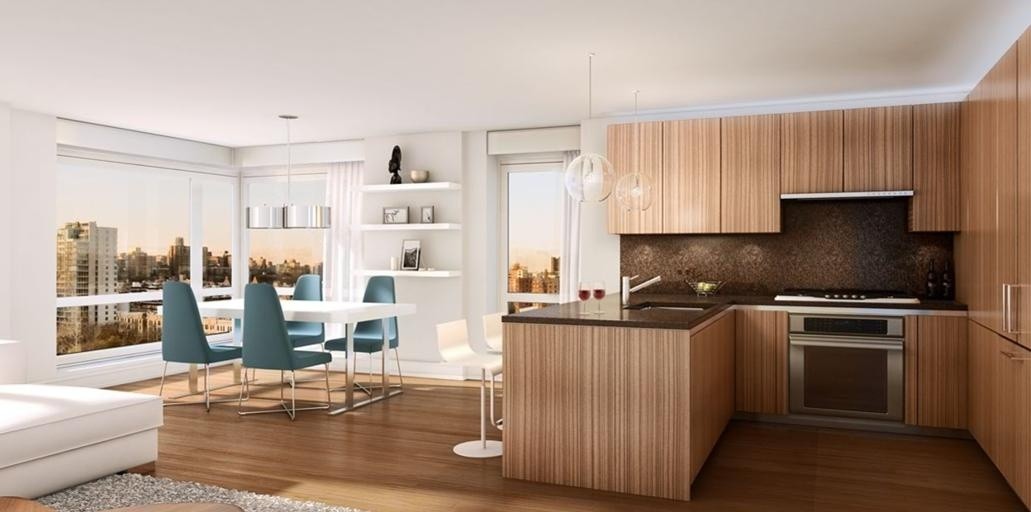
[907,104,965,231]
[358,183,460,279]
[780,105,911,192]
[956,25,1031,508]
[736,312,790,421]
[903,315,966,439]
[609,116,782,233]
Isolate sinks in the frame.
[622,299,719,314]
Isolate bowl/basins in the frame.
[410,170,429,182]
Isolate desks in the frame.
[159,296,416,415]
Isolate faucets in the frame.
[621,273,662,307]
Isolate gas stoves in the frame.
[775,286,918,304]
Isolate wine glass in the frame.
[593,279,605,314]
[578,281,592,316]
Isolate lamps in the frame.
[559,52,654,210]
[245,114,331,228]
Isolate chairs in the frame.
[161,275,404,420]
[437,307,539,458]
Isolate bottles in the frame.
[925,255,938,296]
[940,257,953,296]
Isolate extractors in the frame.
[779,190,916,202]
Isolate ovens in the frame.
[787,313,903,426]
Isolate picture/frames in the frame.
[420,205,435,223]
[401,240,420,270]
[382,207,409,223]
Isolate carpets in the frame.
[35,472,365,512]
